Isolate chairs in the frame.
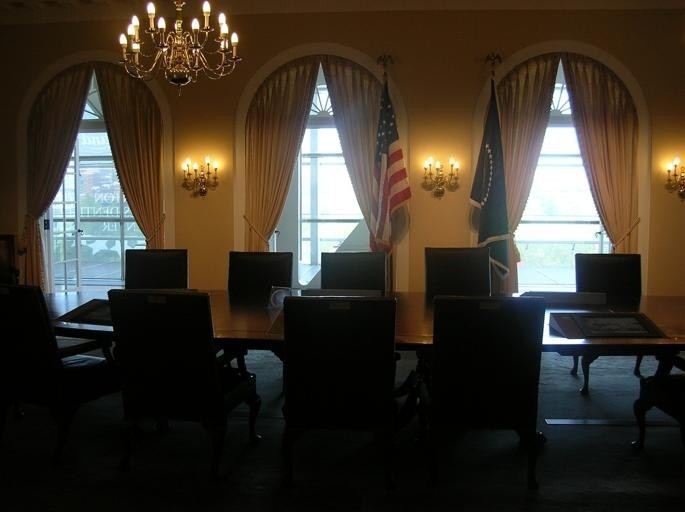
[631,347,685,456]
[0,283,122,473]
[282,296,417,512]
[416,297,547,512]
[425,247,490,293]
[109,290,264,500]
[126,250,187,289]
[571,254,642,394]
[322,253,385,291]
[228,252,293,290]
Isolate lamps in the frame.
[418,153,464,202]
[179,151,224,197]
[117,0,243,97]
[661,155,685,201]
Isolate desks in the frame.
[52,293,685,352]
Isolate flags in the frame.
[369,72,412,244]
[469,75,510,281]
